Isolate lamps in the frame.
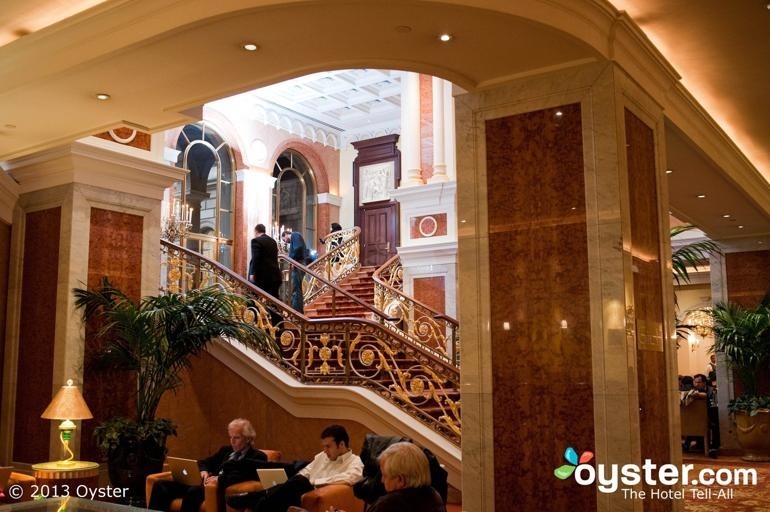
[684,306,714,339]
[40,379,95,468]
[267,221,293,255]
[161,198,193,242]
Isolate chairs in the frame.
[680,392,708,457]
[224,480,365,512]
[146,449,281,512]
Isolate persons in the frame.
[148,417,266,511]
[327,222,343,273]
[278,229,309,318]
[227,421,367,512]
[248,224,286,337]
[367,438,448,512]
[678,351,722,460]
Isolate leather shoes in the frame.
[226,491,256,509]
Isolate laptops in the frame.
[256,468,288,489]
[166,456,208,486]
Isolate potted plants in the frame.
[700,300,770,461]
[71,273,281,500]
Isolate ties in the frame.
[229,452,241,462]
